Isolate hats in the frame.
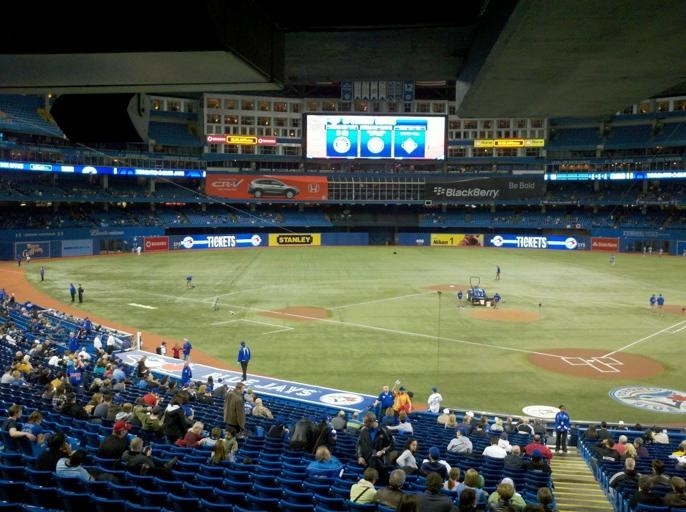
[426,447,440,457]
[113,420,132,431]
[31,425,50,434]
[465,411,473,417]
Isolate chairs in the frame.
[133,369,685,510]
[0,287,133,511]
[0,93,685,236]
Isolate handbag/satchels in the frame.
[401,404,409,413]
[384,446,398,464]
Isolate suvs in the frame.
[248,177,300,199]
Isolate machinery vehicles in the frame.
[467,276,494,308]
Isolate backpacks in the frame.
[156,347,164,355]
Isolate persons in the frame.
[39,266,47,281]
[136,246,142,256]
[268,387,550,512]
[78,282,84,303]
[659,247,664,257]
[22,244,43,257]
[643,246,647,256]
[459,234,481,247]
[649,246,653,256]
[494,265,500,281]
[16,253,21,266]
[582,421,686,511]
[609,253,616,266]
[185,274,194,288]
[683,249,686,256]
[457,288,502,311]
[69,283,76,302]
[0,287,273,481]
[650,294,664,314]
[25,255,30,265]
[555,405,571,454]
[0,151,686,231]
